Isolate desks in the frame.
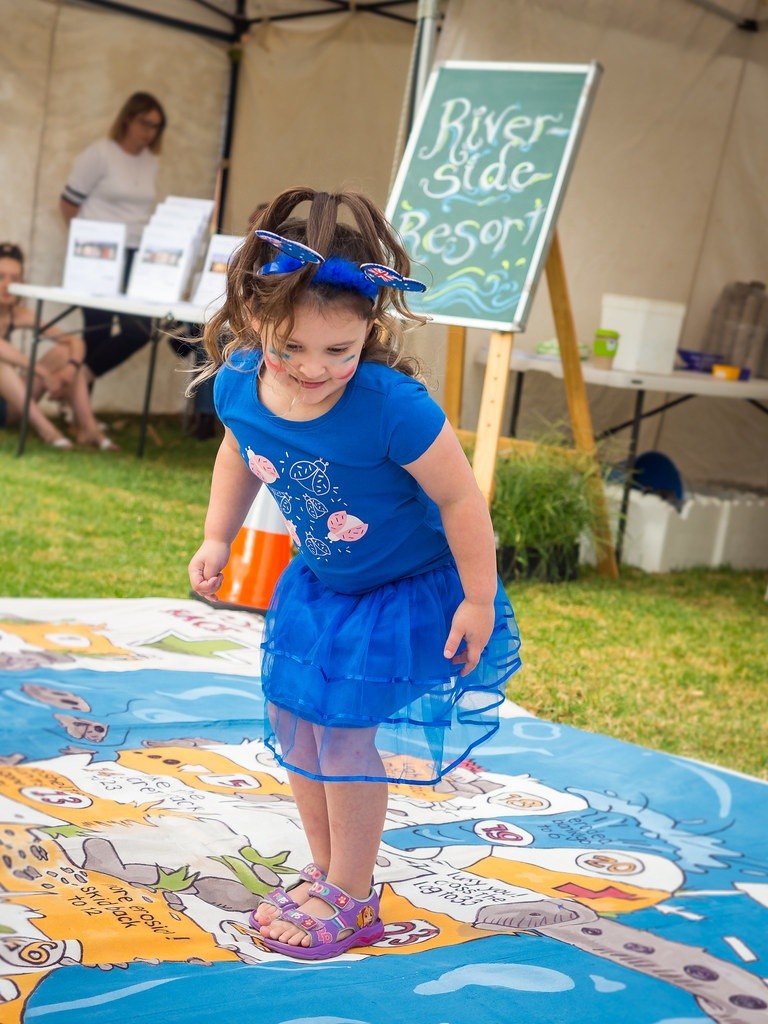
[472,347,768,570]
[7,282,233,460]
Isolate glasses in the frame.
[135,116,162,129]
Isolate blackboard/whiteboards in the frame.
[379,60,606,334]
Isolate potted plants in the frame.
[465,411,649,580]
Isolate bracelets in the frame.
[67,360,80,370]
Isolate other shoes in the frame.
[71,421,121,451]
[53,437,73,448]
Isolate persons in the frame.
[61,92,167,445]
[187,187,521,960]
[0,243,124,451]
[168,204,271,440]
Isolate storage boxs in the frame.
[600,293,685,374]
[579,478,768,573]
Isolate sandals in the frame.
[263,879,385,960]
[249,863,327,933]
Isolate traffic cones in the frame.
[190,485,296,615]
